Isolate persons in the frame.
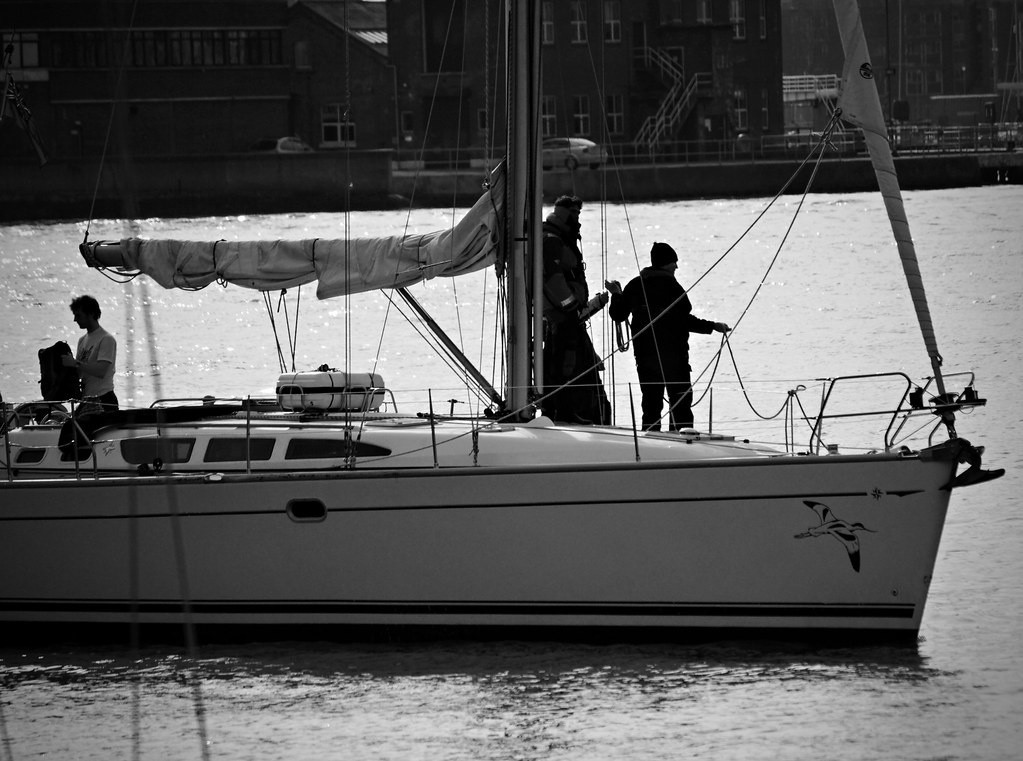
[543,196,609,428]
[62,296,120,461]
[605,241,732,433]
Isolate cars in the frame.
[542,136,608,172]
[238,131,316,152]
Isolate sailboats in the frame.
[0,1,1006,649]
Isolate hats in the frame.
[651,241,678,266]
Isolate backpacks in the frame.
[39,341,82,402]
